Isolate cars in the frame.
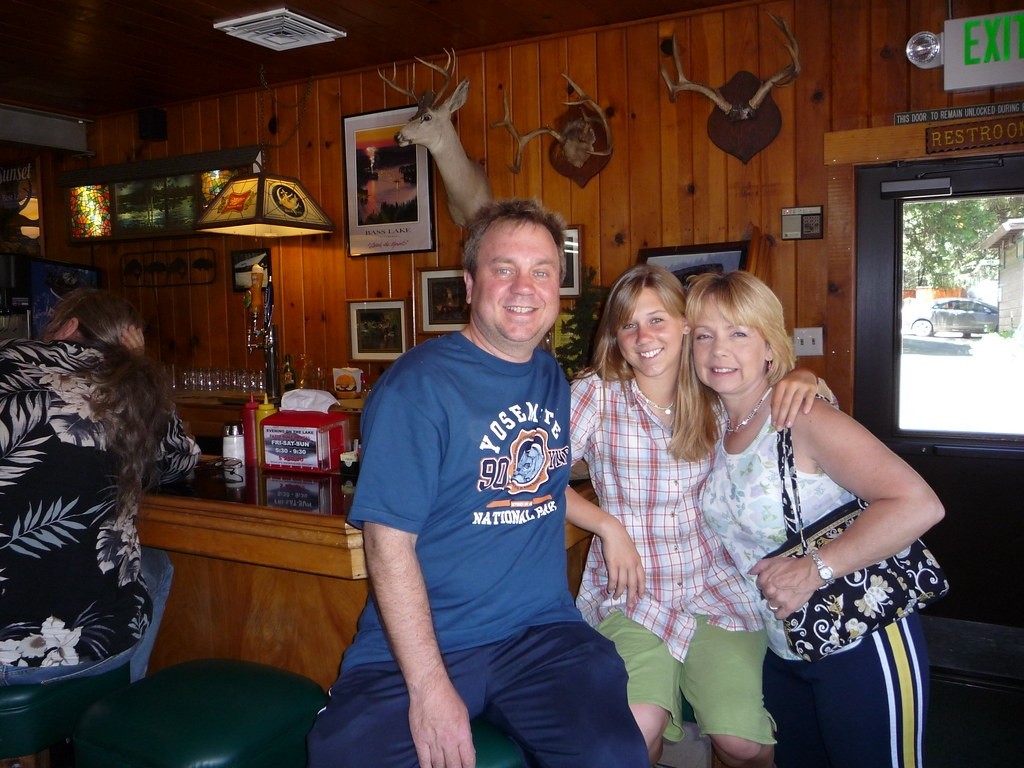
[902,298,999,339]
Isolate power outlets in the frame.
[794,327,823,357]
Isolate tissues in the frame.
[260,388,349,473]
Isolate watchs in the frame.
[808,549,841,591]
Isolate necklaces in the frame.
[717,388,773,434]
[630,377,686,417]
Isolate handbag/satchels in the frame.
[766,431,958,663]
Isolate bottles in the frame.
[283,354,295,392]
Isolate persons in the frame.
[0,279,201,685]
[962,291,975,338]
[305,199,650,768]
[564,263,946,768]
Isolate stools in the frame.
[0,657,728,768]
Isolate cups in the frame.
[180,367,266,390]
[224,467,247,503]
[223,421,246,465]
[312,367,327,392]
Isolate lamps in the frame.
[195,63,336,238]
[904,10,1024,91]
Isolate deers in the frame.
[376,47,492,231]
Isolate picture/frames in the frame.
[413,265,471,336]
[345,298,408,362]
[636,240,751,287]
[230,248,272,292]
[341,103,436,256]
[557,224,582,300]
